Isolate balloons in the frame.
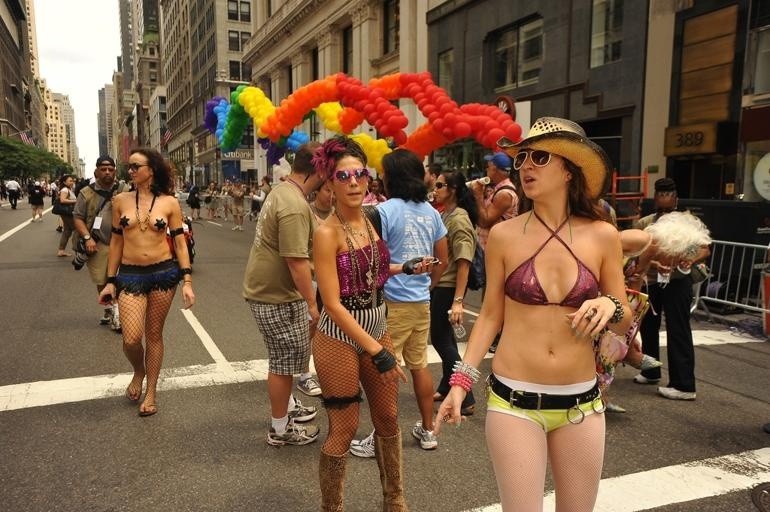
[205,71,521,178]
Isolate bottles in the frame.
[104,296,113,319]
[448,310,466,338]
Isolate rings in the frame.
[584,308,597,321]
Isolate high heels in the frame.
[623,355,662,370]
[606,401,625,413]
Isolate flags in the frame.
[163,129,172,144]
[29,138,35,146]
[20,131,29,144]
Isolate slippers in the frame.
[126,370,145,400]
[58,252,72,257]
[139,402,158,417]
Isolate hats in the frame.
[496,116,612,201]
[96,156,116,166]
[484,152,511,171]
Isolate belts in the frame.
[489,373,600,410]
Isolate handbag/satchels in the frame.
[204,196,211,203]
[52,199,74,216]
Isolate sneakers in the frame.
[350,430,377,458]
[657,385,697,400]
[287,396,318,423]
[232,225,244,231]
[412,419,438,450]
[635,374,661,383]
[99,309,122,330]
[266,415,319,445]
[296,378,322,396]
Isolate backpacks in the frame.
[467,242,484,291]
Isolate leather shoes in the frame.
[433,392,444,401]
[460,406,474,415]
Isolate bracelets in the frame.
[606,295,623,323]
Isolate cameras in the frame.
[71,238,97,270]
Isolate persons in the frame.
[5,151,519,512]
[595,210,711,412]
[433,116,633,512]
[635,178,710,400]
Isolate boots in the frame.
[374,429,408,511]
[319,445,351,511]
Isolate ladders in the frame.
[611,167,648,231]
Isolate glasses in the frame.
[330,169,370,185]
[127,163,148,172]
[513,149,553,170]
[436,181,449,188]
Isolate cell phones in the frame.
[413,257,439,270]
